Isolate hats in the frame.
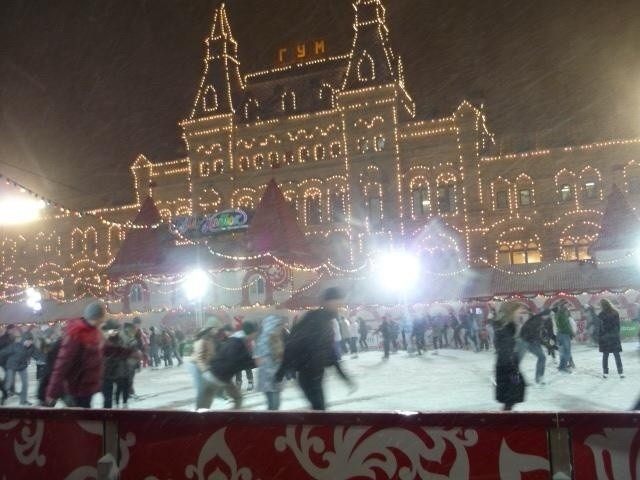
[84,302,105,320]
[23,332,33,339]
[242,322,256,335]
[101,317,141,328]
[204,317,223,328]
[324,287,348,299]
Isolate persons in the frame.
[0,288,154,412]
[190,287,353,410]
[136,325,187,368]
[340,307,491,358]
[489,299,578,411]
[596,297,628,379]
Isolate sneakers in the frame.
[603,374,608,378]
[248,382,253,391]
[620,374,625,378]
